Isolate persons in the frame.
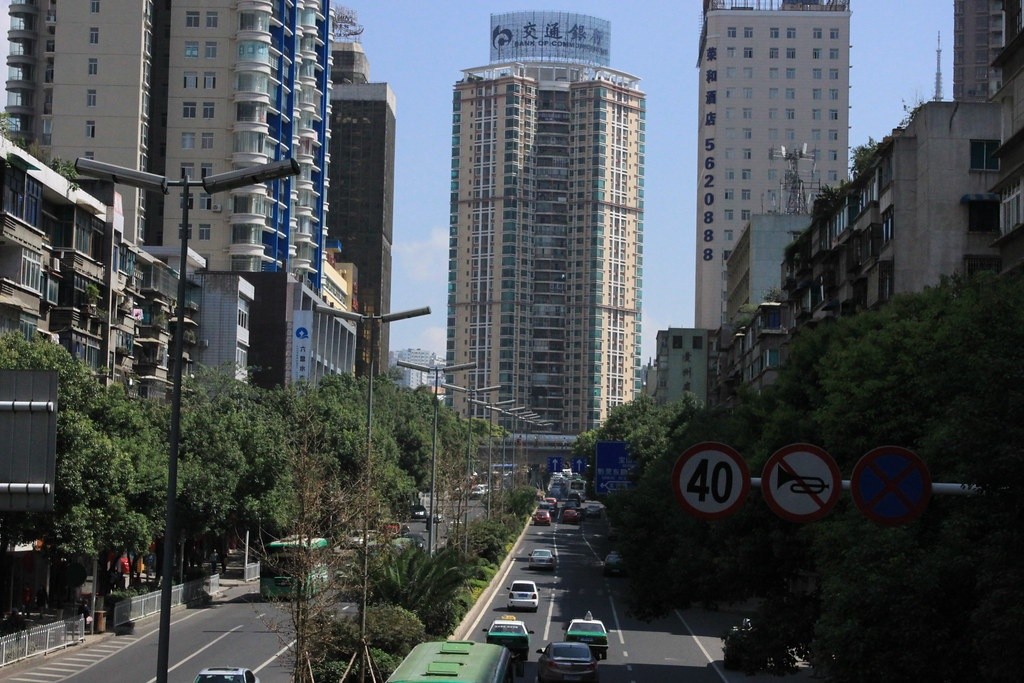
[209,549,228,574]
[22,584,33,619]
[0,608,27,637]
[37,585,48,620]
[115,569,141,588]
[79,599,91,630]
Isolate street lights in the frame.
[439,382,501,563]
[313,304,435,683]
[64,158,303,683]
[394,359,476,561]
[466,398,556,523]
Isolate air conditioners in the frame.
[45,15,54,22]
[197,339,208,347]
[211,203,222,212]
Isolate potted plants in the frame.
[80,283,100,316]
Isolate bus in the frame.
[255,534,330,600]
[381,639,524,683]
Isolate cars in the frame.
[532,509,551,526]
[562,611,611,659]
[339,522,426,551]
[539,468,586,513]
[585,505,602,518]
[536,642,600,683]
[482,615,535,660]
[450,482,505,505]
[190,666,261,683]
[571,507,585,521]
[428,510,444,522]
[411,505,427,519]
[562,510,577,524]
[506,579,541,613]
[528,549,556,571]
[602,551,629,577]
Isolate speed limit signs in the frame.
[672,441,751,521]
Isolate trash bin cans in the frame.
[94,611,107,632]
